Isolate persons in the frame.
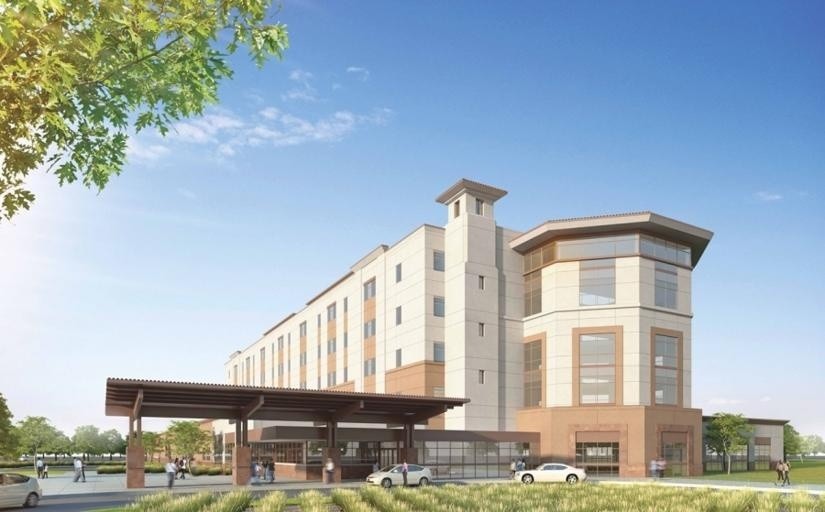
[36,456,44,478]
[174,456,186,479]
[73,457,86,482]
[164,458,177,490]
[775,458,785,486]
[510,457,526,480]
[401,457,409,487]
[373,459,380,472]
[252,456,274,484]
[650,456,668,482]
[325,457,335,484]
[42,462,48,479]
[781,458,792,486]
[72,456,77,473]
